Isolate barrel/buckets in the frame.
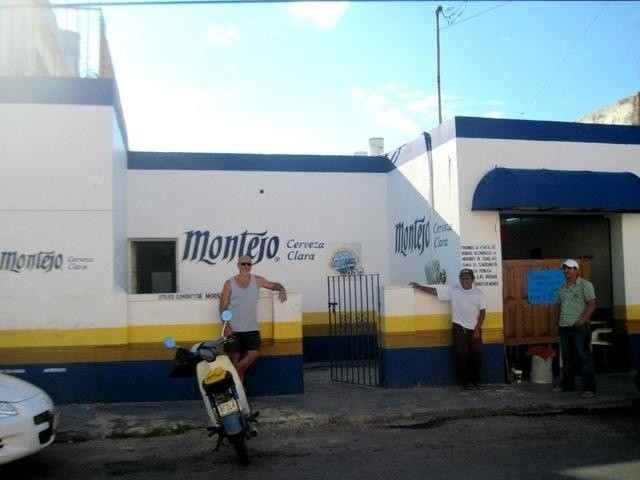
[531,354,554,384]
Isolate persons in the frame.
[217,255,288,385]
[407,267,487,391]
[549,258,599,398]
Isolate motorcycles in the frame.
[161,310,265,469]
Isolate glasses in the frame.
[240,262,251,266]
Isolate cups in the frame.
[514,370,523,382]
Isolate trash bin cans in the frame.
[530,350,556,384]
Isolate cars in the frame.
[1,367,61,466]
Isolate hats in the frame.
[458,269,474,277]
[561,258,580,269]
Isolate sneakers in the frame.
[581,391,594,397]
[552,385,561,391]
[466,383,484,390]
